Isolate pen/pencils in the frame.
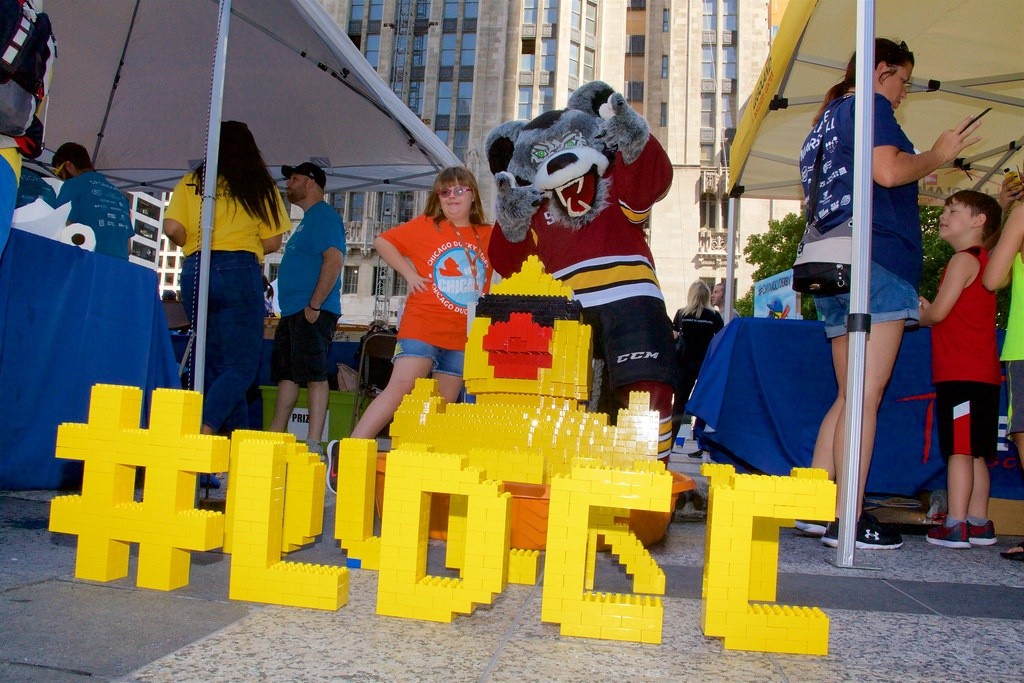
[960,107,992,133]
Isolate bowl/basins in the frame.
[375,452,698,552]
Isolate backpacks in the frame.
[0,0,58,159]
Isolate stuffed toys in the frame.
[0,0,58,250]
[487,78,672,471]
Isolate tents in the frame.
[38,1,469,420]
[719,0,1024,319]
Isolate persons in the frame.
[798,39,982,550]
[916,190,1001,549]
[669,277,742,458]
[52,142,134,261]
[326,166,492,497]
[163,120,346,488]
[983,170,1024,561]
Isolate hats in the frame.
[281,162,326,189]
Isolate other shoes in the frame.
[306,439,326,461]
[327,440,340,494]
[199,474,222,488]
[1001,542,1024,561]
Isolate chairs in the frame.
[352,332,398,431]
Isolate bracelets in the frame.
[308,303,322,313]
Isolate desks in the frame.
[0,228,184,492]
[687,314,1024,537]
[173,335,361,436]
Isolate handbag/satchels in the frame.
[793,216,853,294]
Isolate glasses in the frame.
[884,41,909,58]
[55,161,70,180]
[437,186,472,198]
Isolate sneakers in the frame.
[926,513,997,549]
[687,449,703,461]
[795,508,903,549]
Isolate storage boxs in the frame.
[987,497,1024,535]
[258,385,375,455]
